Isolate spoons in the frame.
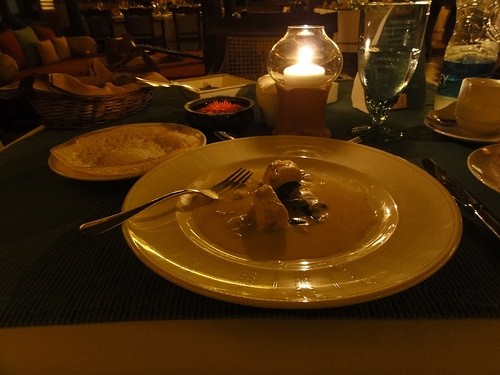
[425,113,455,127]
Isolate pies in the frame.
[49,124,205,175]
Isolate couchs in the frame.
[0,25,108,99]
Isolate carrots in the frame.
[195,99,244,113]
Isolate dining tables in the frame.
[0,76,500,375]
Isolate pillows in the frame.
[13,25,43,66]
[0,29,27,69]
[32,21,56,40]
[53,36,71,58]
[35,40,60,65]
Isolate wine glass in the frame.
[349,0,432,139]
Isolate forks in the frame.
[80,167,254,237]
[213,131,235,141]
[349,136,365,145]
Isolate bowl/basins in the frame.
[185,96,256,134]
[172,73,255,99]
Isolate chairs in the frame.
[120,6,168,48]
[82,9,115,50]
[170,7,202,50]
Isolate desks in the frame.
[112,11,203,50]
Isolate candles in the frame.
[284,45,327,85]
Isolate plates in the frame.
[467,143,500,193]
[424,111,500,144]
[48,123,207,182]
[121,135,463,309]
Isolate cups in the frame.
[455,77,500,137]
[435,1,500,78]
[256,73,281,128]
[96,0,201,15]
[327,82,339,103]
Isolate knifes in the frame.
[420,157,500,245]
